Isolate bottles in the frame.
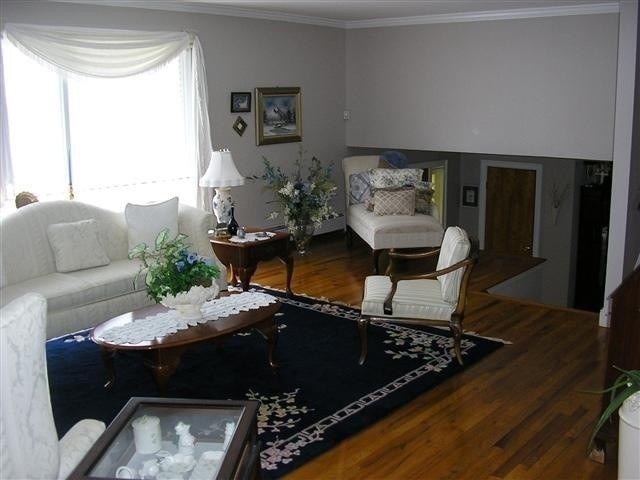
[132,414,161,456]
[227,205,239,236]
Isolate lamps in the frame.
[199,149,244,237]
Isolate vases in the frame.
[294,220,315,257]
[160,278,219,319]
[551,207,561,225]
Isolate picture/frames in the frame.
[461,184,479,208]
[231,87,302,146]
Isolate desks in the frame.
[65,397,260,480]
[210,227,294,298]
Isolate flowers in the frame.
[548,183,571,208]
[130,229,220,303]
[247,151,344,240]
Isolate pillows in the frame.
[47,220,110,273]
[349,152,433,216]
[125,197,179,258]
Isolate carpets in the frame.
[46,282,512,480]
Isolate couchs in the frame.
[0,200,227,340]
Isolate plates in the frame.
[156,451,223,480]
[208,227,276,243]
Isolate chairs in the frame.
[342,155,445,274]
[357,225,480,367]
